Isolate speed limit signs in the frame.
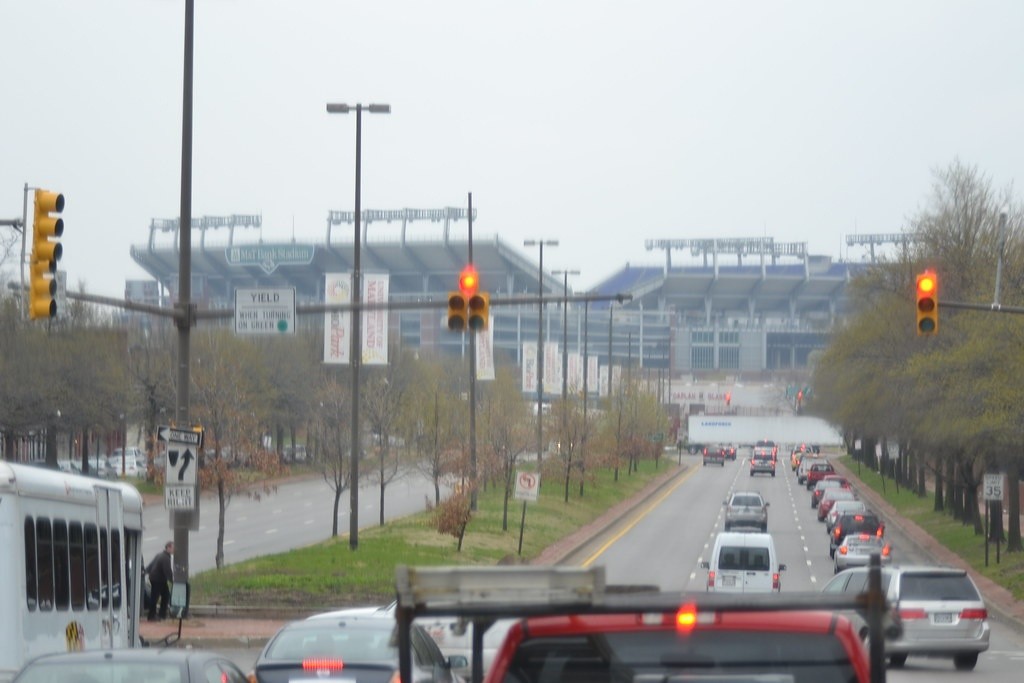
[982,471,1005,501]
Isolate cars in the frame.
[789,436,993,672]
[283,444,307,463]
[8,602,398,682]
[58,444,149,481]
[700,438,777,476]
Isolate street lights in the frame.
[327,104,395,542]
[552,271,578,425]
[524,239,559,455]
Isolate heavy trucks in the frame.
[687,414,846,455]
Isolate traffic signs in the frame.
[157,426,200,448]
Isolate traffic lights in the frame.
[32,184,68,323]
[915,270,940,338]
[445,264,492,333]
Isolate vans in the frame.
[699,531,787,593]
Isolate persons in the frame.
[146,541,174,622]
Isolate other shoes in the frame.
[147,617,167,622]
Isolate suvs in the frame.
[724,492,770,530]
